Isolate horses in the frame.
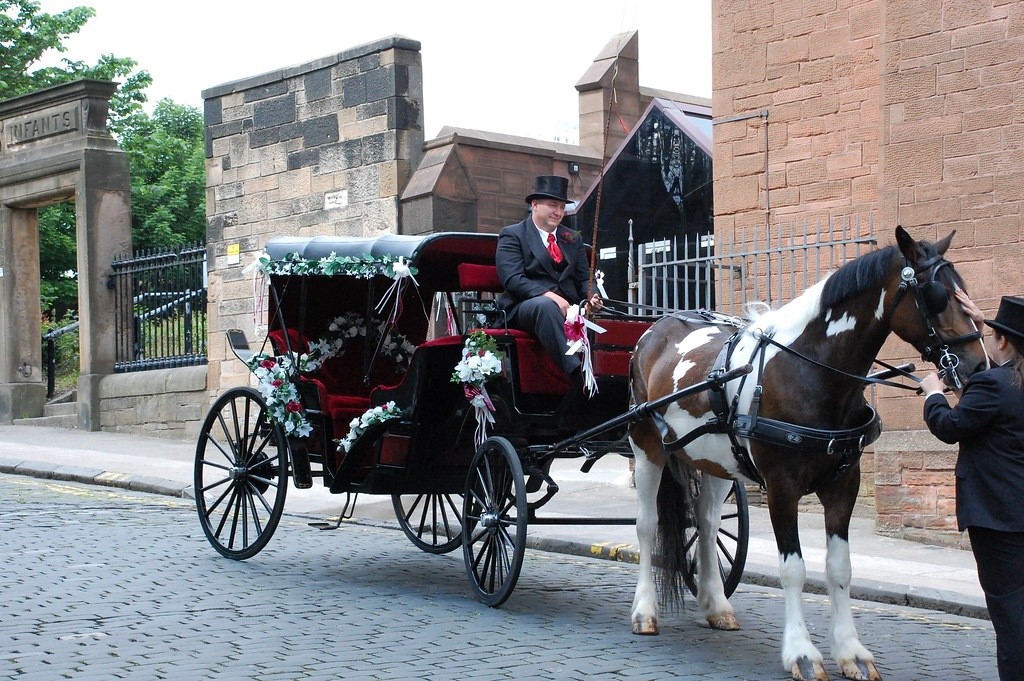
[628,225,990,681]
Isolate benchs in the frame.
[453,263,653,395]
[268,327,428,421]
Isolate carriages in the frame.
[194,230,999,680]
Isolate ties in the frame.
[546,233,563,264]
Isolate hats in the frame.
[984,295,1024,338]
[525,175,575,204]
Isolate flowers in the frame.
[245,308,418,438]
[450,328,507,385]
[240,247,419,281]
[330,396,405,454]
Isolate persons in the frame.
[496,176,603,395]
[919,289,1024,681]
